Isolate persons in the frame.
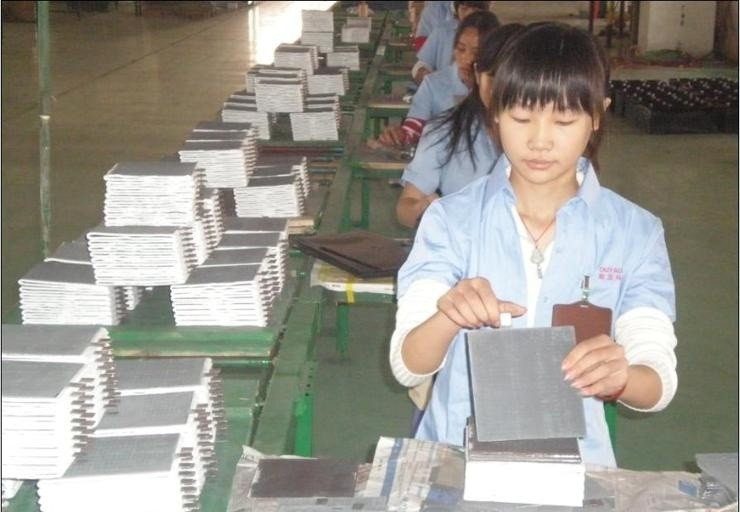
[415,0,455,51]
[388,22,679,468]
[379,11,501,146]
[411,0,491,87]
[395,22,527,228]
